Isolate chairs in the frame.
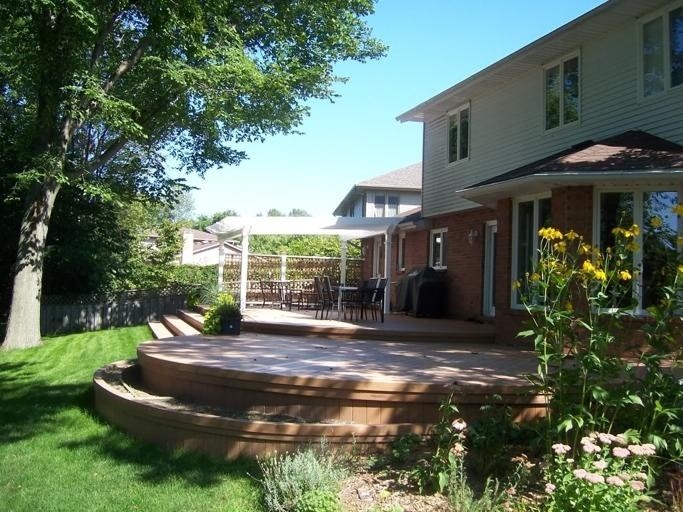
[257,276,388,324]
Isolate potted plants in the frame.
[216,303,242,333]
